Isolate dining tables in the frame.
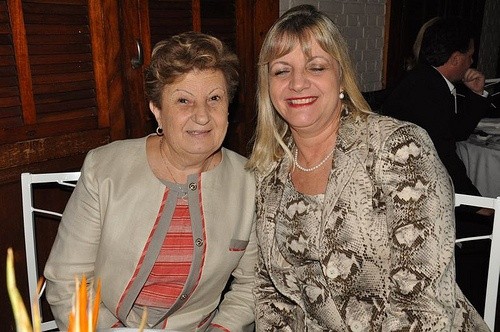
[455,116,500,216]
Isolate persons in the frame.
[41,31,258,331]
[378,16,500,313]
[244,4,493,332]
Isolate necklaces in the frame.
[293,143,336,172]
[159,135,215,203]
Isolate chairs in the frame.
[455,190,500,332]
[20,171,81,332]
